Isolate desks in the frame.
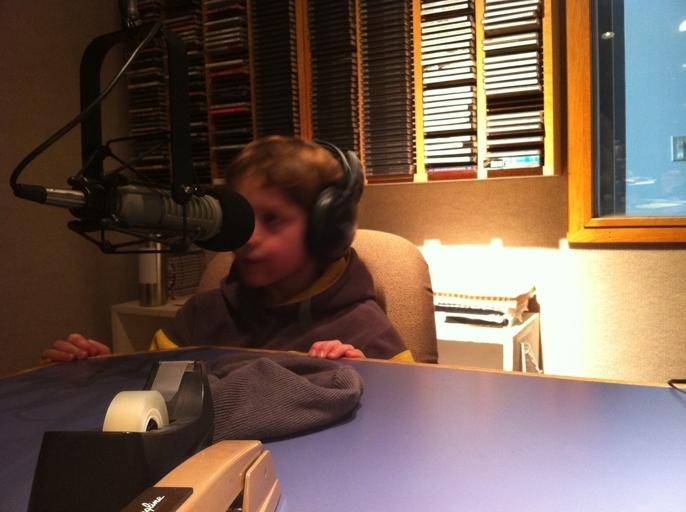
[0,343,686,512]
[112,293,541,373]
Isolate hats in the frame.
[205,351,365,446]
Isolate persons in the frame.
[38,135,416,368]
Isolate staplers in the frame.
[121,439,282,512]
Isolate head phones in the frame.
[308,138,366,264]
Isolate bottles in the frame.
[138,233,169,308]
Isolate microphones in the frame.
[104,183,256,252]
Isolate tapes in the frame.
[103,360,189,432]
